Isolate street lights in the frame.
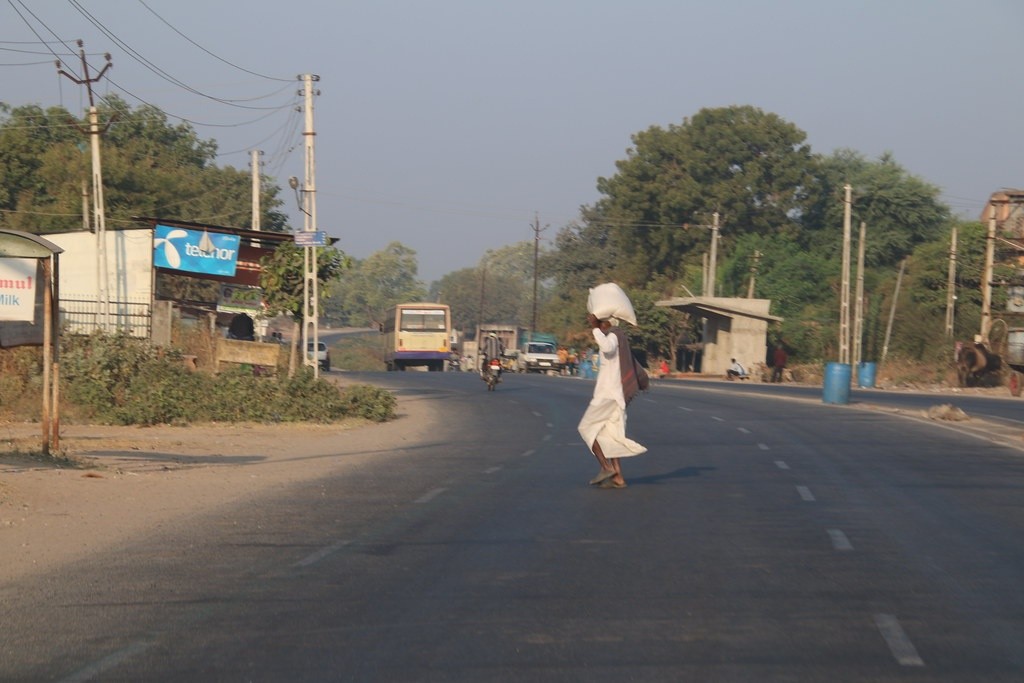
[476,260,502,372]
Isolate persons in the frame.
[578,313,648,488]
[448,348,517,373]
[556,343,593,377]
[771,344,786,382]
[271,331,287,345]
[655,358,670,380]
[482,331,506,383]
[727,358,745,380]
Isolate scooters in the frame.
[478,347,506,392]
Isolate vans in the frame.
[306,342,331,372]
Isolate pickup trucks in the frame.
[516,341,561,374]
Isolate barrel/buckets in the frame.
[823,360,853,404]
[858,361,876,387]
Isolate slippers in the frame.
[589,469,617,485]
[596,479,626,488]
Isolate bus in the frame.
[378,303,452,373]
